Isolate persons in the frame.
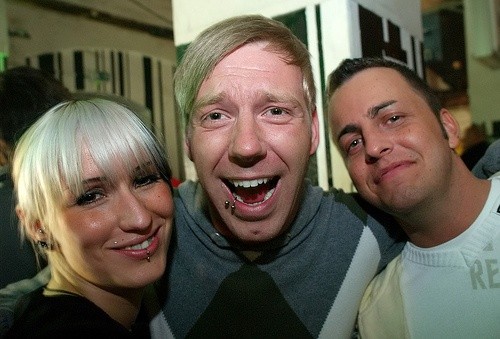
[6,97,175,339]
[460,121,500,179]
[326,56,500,339]
[0,67,74,289]
[0,14,499,339]
